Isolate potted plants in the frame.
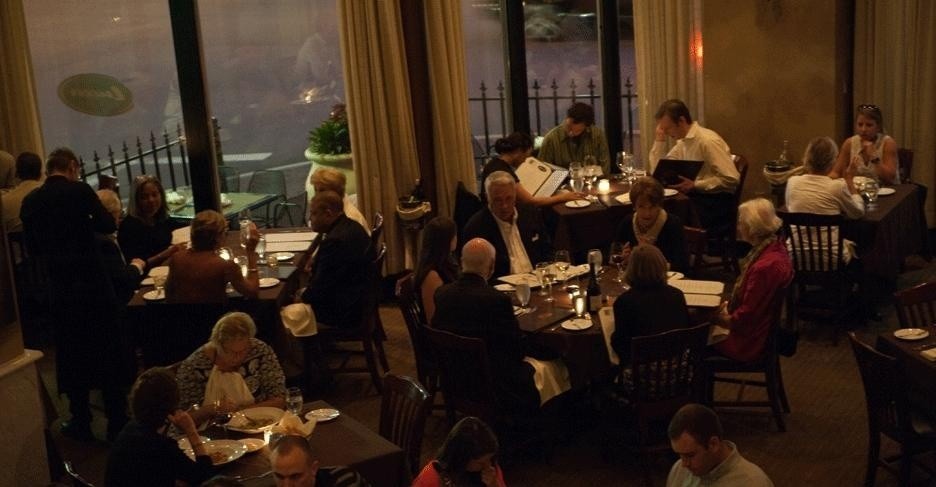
[304,103,361,232]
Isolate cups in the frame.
[864,175,879,207]
[635,167,647,179]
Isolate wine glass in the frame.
[238,207,252,232]
[267,253,277,275]
[588,249,602,280]
[253,237,266,264]
[584,156,597,186]
[286,386,303,415]
[556,249,570,291]
[212,398,232,437]
[516,280,531,308]
[573,288,588,317]
[611,246,624,282]
[570,162,584,195]
[621,155,635,183]
[615,152,628,176]
[537,262,557,302]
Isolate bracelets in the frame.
[248,269,258,273]
[192,443,202,453]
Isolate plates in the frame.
[561,319,593,330]
[260,278,280,288]
[839,177,874,189]
[880,187,895,195]
[143,289,165,299]
[225,409,285,432]
[271,251,294,261]
[305,409,340,420]
[183,438,247,464]
[178,437,211,449]
[895,328,929,340]
[566,200,592,207]
[239,439,264,451]
[148,265,169,277]
[665,272,684,278]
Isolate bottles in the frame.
[779,139,791,163]
[587,261,603,315]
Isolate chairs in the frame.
[893,280,935,330]
[704,270,800,434]
[678,224,708,279]
[218,165,241,230]
[318,212,391,345]
[240,171,295,232]
[303,241,388,398]
[847,330,936,486]
[602,319,712,476]
[693,152,749,274]
[320,370,433,487]
[775,209,869,345]
[398,270,443,395]
[420,323,573,463]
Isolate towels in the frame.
[200,364,255,422]
[280,303,319,338]
[711,300,730,336]
[524,357,572,407]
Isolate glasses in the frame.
[857,104,880,110]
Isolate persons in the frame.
[785,137,883,322]
[536,103,611,174]
[666,404,775,487]
[0,150,15,195]
[202,476,244,487]
[461,171,553,279]
[295,171,371,354]
[431,237,556,454]
[104,368,213,487]
[117,176,187,275]
[599,244,691,465]
[19,148,131,442]
[608,178,692,274]
[480,133,585,209]
[412,417,506,487]
[164,211,260,304]
[95,190,145,303]
[286,192,369,398]
[414,218,457,326]
[166,312,284,441]
[828,104,900,185]
[270,436,373,487]
[1,152,45,265]
[648,99,740,226]
[706,198,792,409]
